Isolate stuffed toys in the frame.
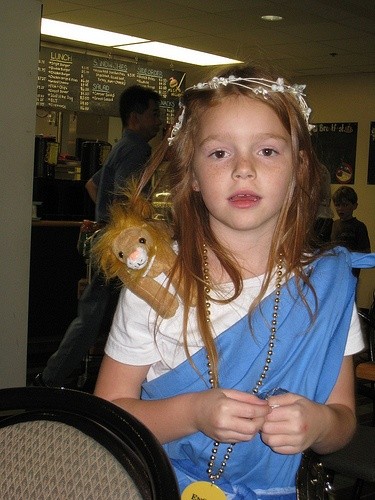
[84,182,201,317]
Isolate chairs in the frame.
[299,308,375,500]
[0,387,181,500]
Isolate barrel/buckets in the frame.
[81,142,106,180]
[35,137,56,178]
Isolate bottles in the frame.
[78,220,92,256]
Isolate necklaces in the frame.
[178,233,285,500]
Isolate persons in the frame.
[32,85,164,388]
[330,186,372,304]
[91,66,365,500]
[312,151,333,243]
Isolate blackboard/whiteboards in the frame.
[36,45,186,131]
[366,121,375,185]
[308,122,358,184]
